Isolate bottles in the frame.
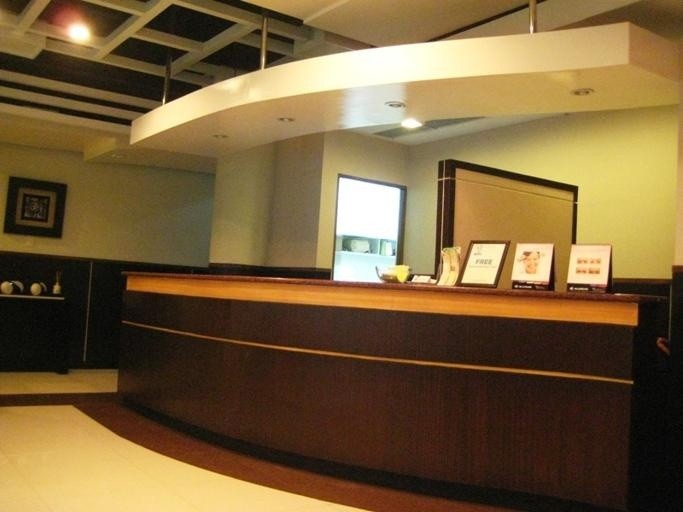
[53,281,62,294]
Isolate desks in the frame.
[0,294,66,375]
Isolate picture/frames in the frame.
[456,240,510,288]
[2,174,69,238]
